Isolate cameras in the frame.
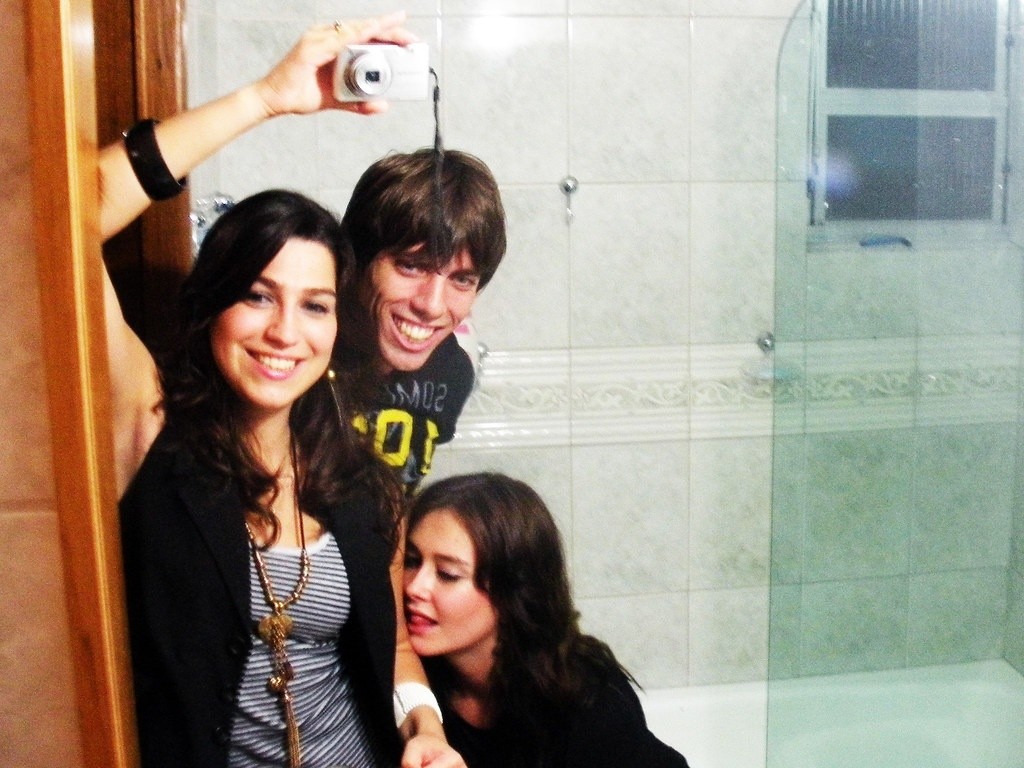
[331,41,430,103]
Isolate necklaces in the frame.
[244,425,311,768]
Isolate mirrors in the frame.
[0,1,1023,768]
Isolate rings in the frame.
[334,20,343,32]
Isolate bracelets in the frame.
[393,681,443,727]
[122,118,187,203]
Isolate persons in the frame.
[98,12,689,768]
